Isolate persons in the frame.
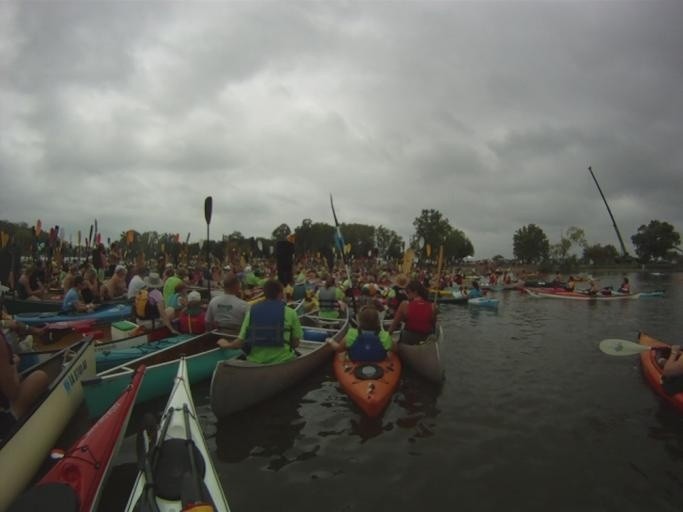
[448,263,634,298]
[0,241,450,367]
[1,329,51,441]
[661,341,683,379]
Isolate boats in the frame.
[640,330,683,415]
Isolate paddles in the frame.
[600,338,683,357]
[137,413,161,512]
[1,190,445,312]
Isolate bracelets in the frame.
[671,351,678,355]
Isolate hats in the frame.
[187,289,202,303]
[142,272,164,288]
